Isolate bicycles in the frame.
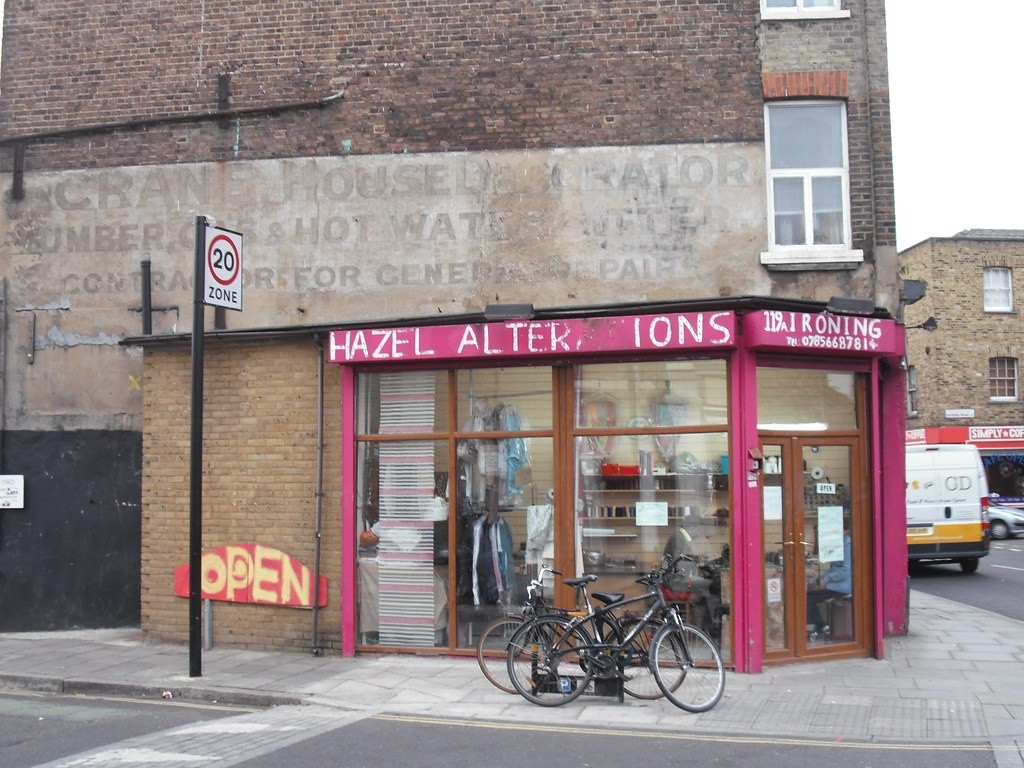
[476,553,726,713]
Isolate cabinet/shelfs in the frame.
[581,472,728,541]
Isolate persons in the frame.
[806,517,851,641]
[662,514,722,642]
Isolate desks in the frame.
[586,560,650,641]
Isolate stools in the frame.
[826,595,854,642]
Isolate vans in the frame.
[905,443,992,574]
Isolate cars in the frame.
[988,499,1024,539]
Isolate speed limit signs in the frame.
[202,226,246,315]
